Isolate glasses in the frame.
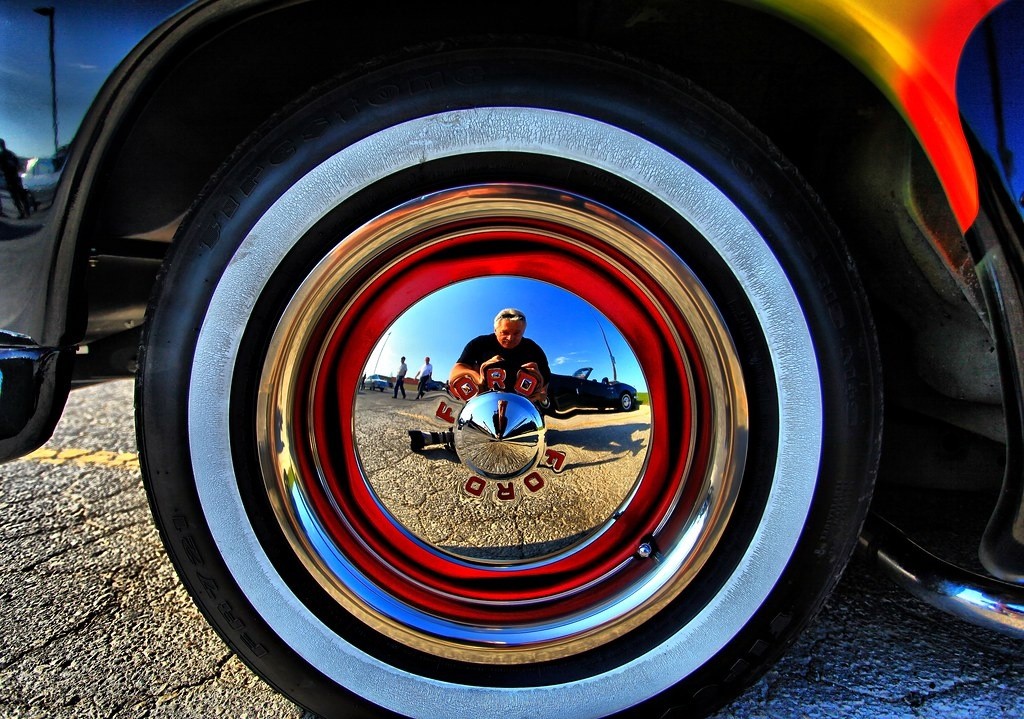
[497,314,526,322]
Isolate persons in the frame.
[360,374,366,390]
[451,309,551,401]
[493,400,508,438]
[0,140,31,219]
[391,357,408,399]
[414,357,432,400]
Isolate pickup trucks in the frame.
[363,375,387,392]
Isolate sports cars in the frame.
[534,367,637,416]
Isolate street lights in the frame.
[32,6,59,148]
